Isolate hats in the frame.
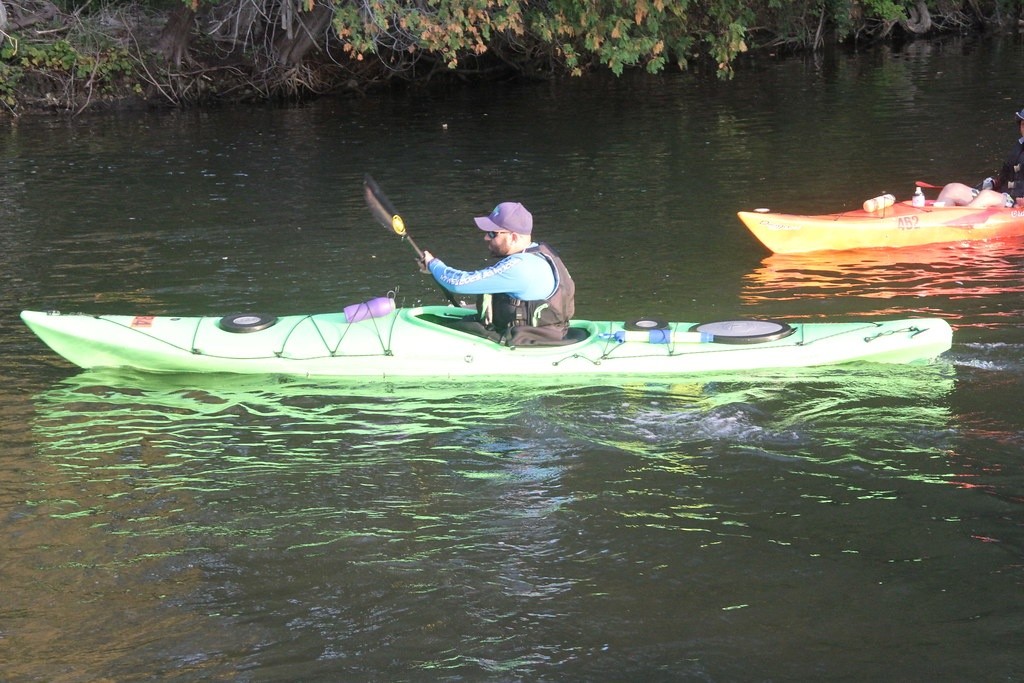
[1016,108,1024,119]
[473,201,533,236]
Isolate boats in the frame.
[737,196,1024,256]
[18,305,954,377]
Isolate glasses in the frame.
[487,230,513,239]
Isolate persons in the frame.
[414,200,575,346]
[936,106,1024,210]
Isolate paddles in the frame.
[362,172,465,311]
[913,178,986,190]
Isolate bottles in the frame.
[344,290,396,322]
[863,193,896,212]
[911,187,925,208]
